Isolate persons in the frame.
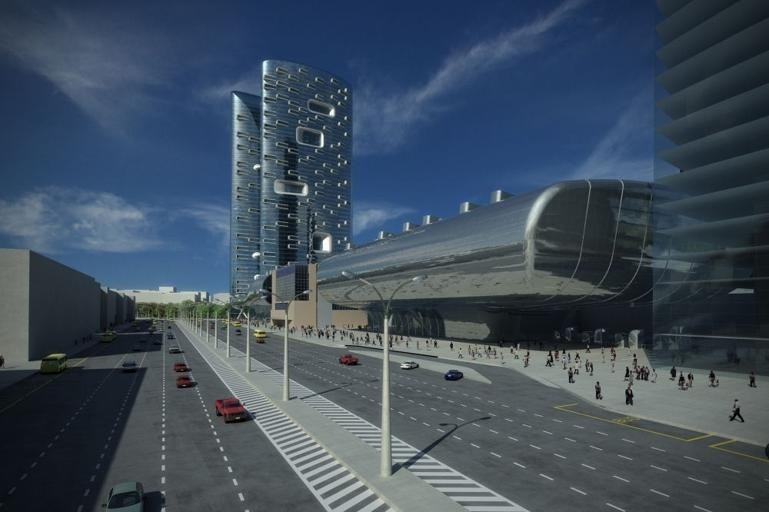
[594,352,658,406]
[390,334,438,352]
[670,365,716,388]
[545,344,617,384]
[288,322,382,353]
[729,398,745,422]
[449,340,529,368]
[749,371,757,387]
